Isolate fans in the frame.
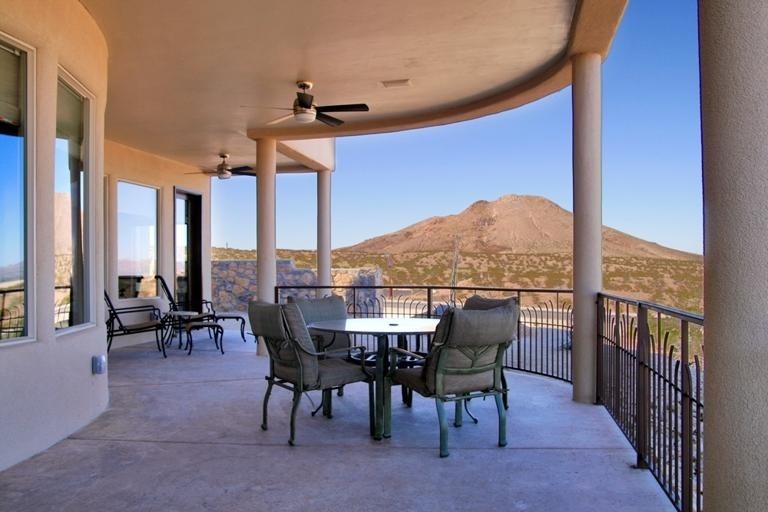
[184,153,259,180]
[240,82,369,128]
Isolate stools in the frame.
[183,312,247,356]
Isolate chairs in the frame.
[102,275,221,358]
